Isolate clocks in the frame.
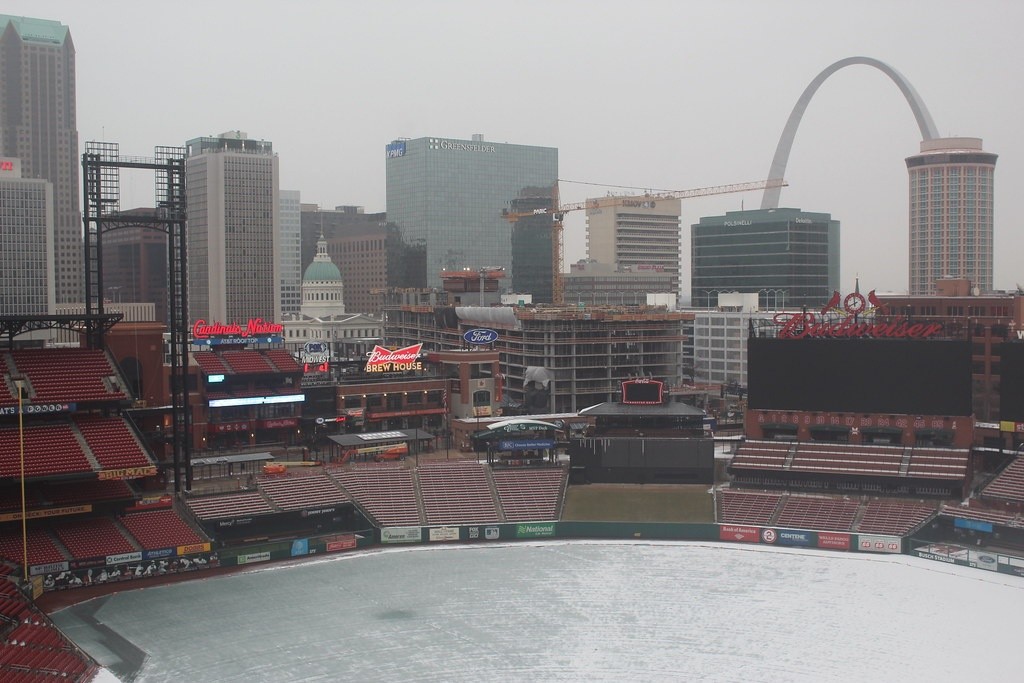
[844,294,865,314]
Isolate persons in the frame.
[42,552,221,593]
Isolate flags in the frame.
[441,379,449,422]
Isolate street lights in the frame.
[16,384,32,583]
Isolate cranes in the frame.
[500,175,788,307]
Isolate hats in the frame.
[48,574,53,577]
[151,560,155,562]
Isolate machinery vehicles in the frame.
[261,460,322,474]
[339,442,409,465]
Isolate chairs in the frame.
[722,441,1024,537]
[193,349,302,375]
[0,416,564,682]
[0,348,127,406]
